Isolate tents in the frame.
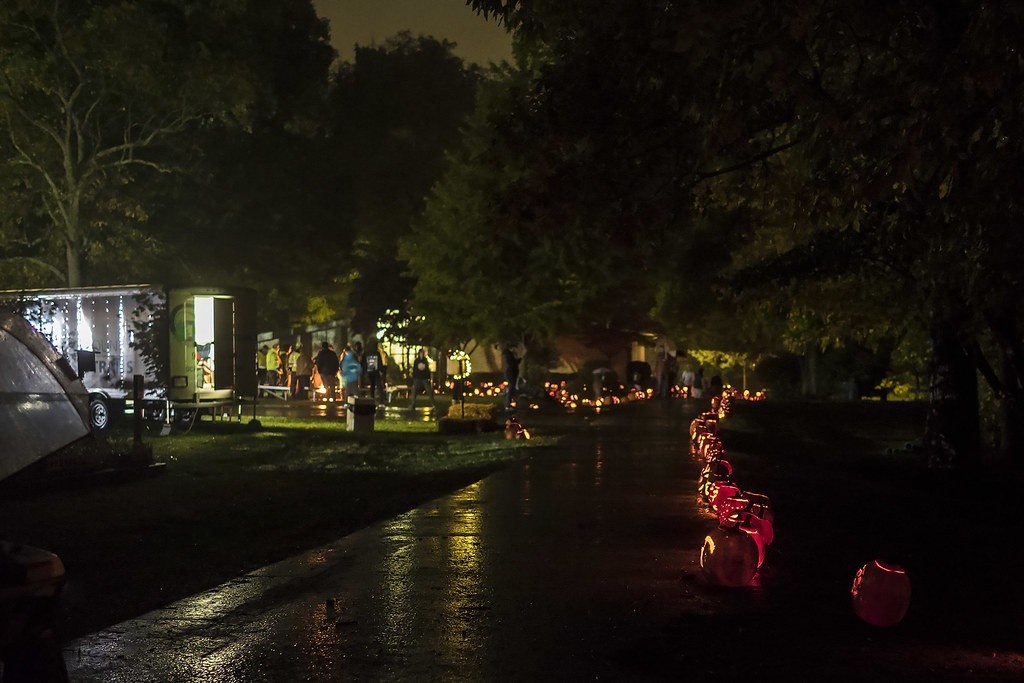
[0,305,105,495]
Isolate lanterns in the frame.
[686,388,911,628]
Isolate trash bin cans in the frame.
[346,396,375,433]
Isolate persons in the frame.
[256,336,436,411]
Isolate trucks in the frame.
[0,283,259,435]
